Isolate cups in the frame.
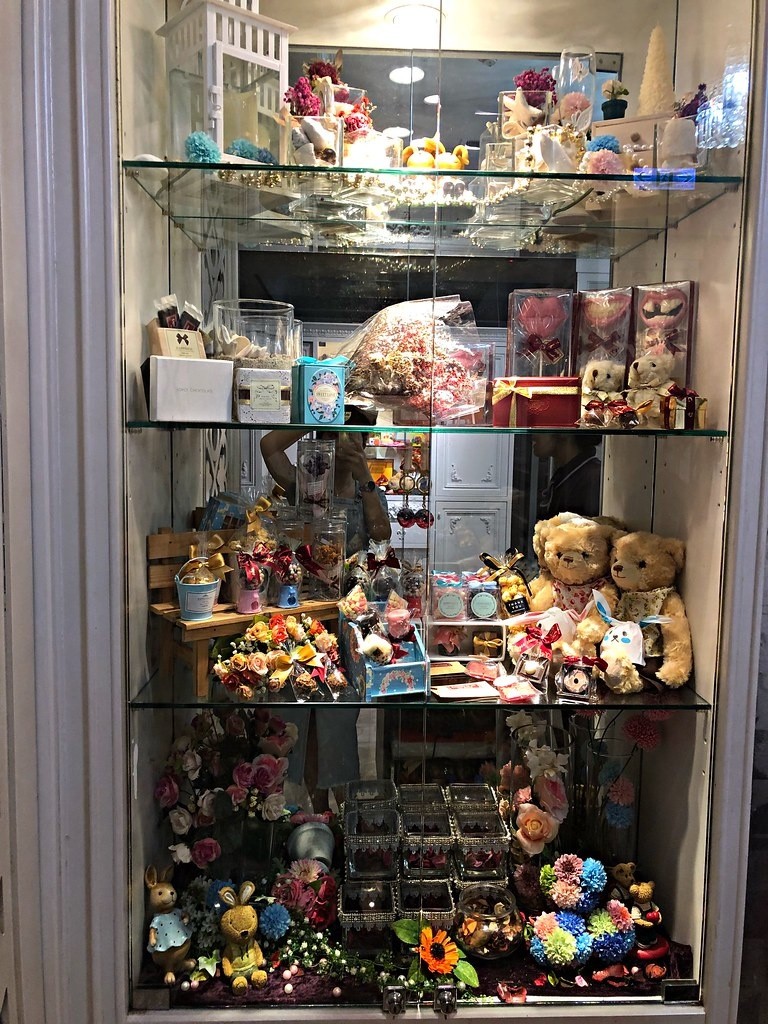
[557,45,596,146]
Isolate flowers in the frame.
[154,707,338,976]
[224,138,278,166]
[392,919,480,988]
[585,134,623,174]
[283,48,377,144]
[155,130,220,199]
[320,295,488,421]
[499,709,685,972]
[512,67,590,127]
[210,612,348,703]
[602,79,630,99]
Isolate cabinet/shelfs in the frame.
[591,112,672,174]
[365,433,514,571]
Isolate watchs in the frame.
[359,480,375,493]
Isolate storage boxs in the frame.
[337,779,512,962]
[491,376,582,429]
[365,459,393,486]
[140,317,346,425]
[428,570,506,686]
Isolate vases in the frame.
[510,724,575,913]
[586,737,642,868]
[284,822,336,866]
[600,100,628,120]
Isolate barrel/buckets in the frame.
[174,557,219,620]
[241,315,303,360]
[212,299,293,357]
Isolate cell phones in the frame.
[339,431,359,450]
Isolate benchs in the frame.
[147,520,351,698]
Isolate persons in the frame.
[260,404,391,814]
[528,433,601,516]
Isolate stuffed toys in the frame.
[218,881,268,996]
[145,863,197,985]
[507,512,693,695]
[611,862,662,948]
[579,345,676,431]
